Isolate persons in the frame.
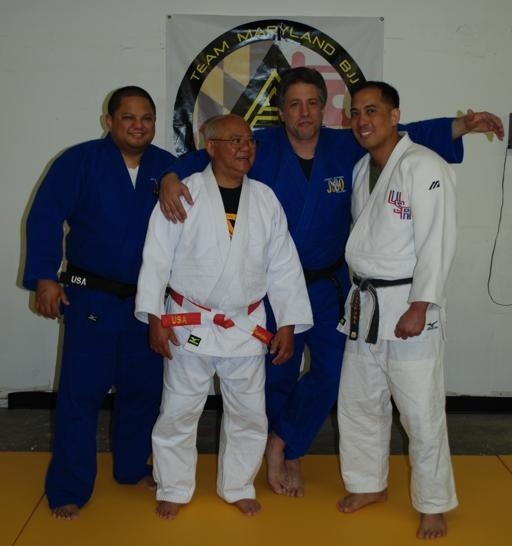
[334,82,460,540]
[133,113,314,520]
[154,66,504,498]
[21,84,178,521]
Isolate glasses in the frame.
[208,136,257,147]
